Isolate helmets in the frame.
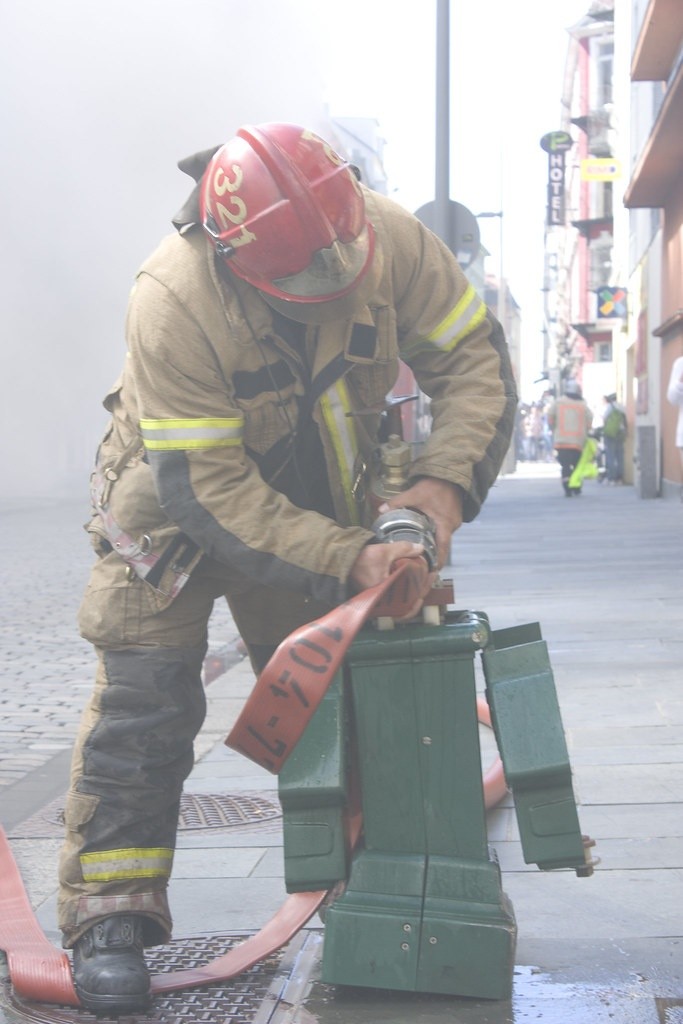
[201,122,377,298]
[563,379,585,396]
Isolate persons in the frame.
[666,355,683,448]
[59,122,519,1008]
[519,382,628,496]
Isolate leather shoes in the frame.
[70,912,155,1015]
